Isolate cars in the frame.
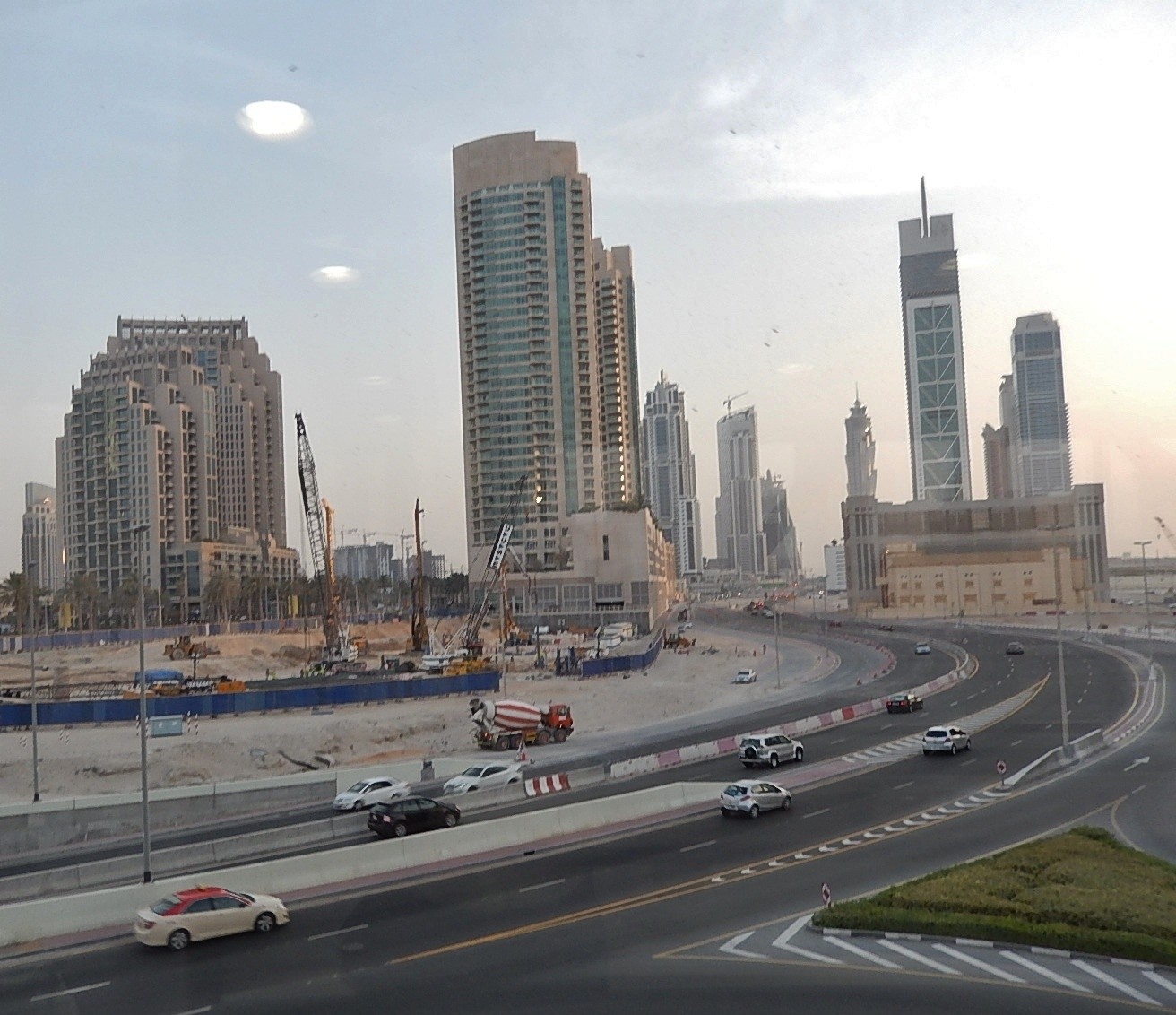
[828,619,842,627]
[735,669,757,684]
[332,776,410,812]
[743,604,777,619]
[878,623,891,630]
[1006,642,1025,655]
[720,779,794,820]
[443,764,524,796]
[132,881,292,951]
[916,642,931,655]
[886,694,924,712]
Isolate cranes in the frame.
[1155,515,1176,554]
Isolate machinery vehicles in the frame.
[466,698,575,752]
[162,634,208,660]
[289,410,532,680]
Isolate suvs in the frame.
[921,725,972,757]
[738,732,805,770]
[367,795,462,840]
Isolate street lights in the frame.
[132,522,160,883]
[821,574,831,659]
[27,559,44,802]
[1038,524,1073,745]
[1133,539,1153,638]
